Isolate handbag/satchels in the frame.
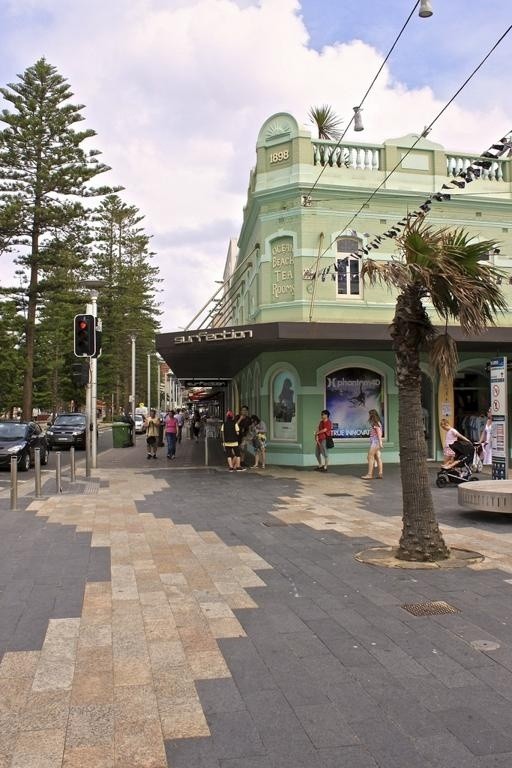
[470,446,484,474]
[146,435,157,445]
[327,436,334,450]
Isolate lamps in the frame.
[418,0,433,18]
[352,106,364,132]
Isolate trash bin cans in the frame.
[111,416,135,448]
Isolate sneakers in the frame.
[377,471,383,480]
[361,475,374,480]
[166,454,176,460]
[235,467,247,473]
[315,465,328,473]
[147,453,157,460]
[228,467,234,472]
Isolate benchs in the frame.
[457,478,512,519]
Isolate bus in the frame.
[135,402,158,419]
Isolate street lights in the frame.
[124,328,144,426]
[146,350,179,416]
[76,279,110,470]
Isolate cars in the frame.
[45,412,100,450]
[0,419,49,469]
[124,409,188,434]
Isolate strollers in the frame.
[434,438,488,491]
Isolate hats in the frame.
[225,410,235,419]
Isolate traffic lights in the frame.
[68,362,84,387]
[72,314,95,356]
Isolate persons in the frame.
[316,410,331,470]
[360,410,388,478]
[440,418,472,460]
[479,411,493,463]
[145,404,269,473]
